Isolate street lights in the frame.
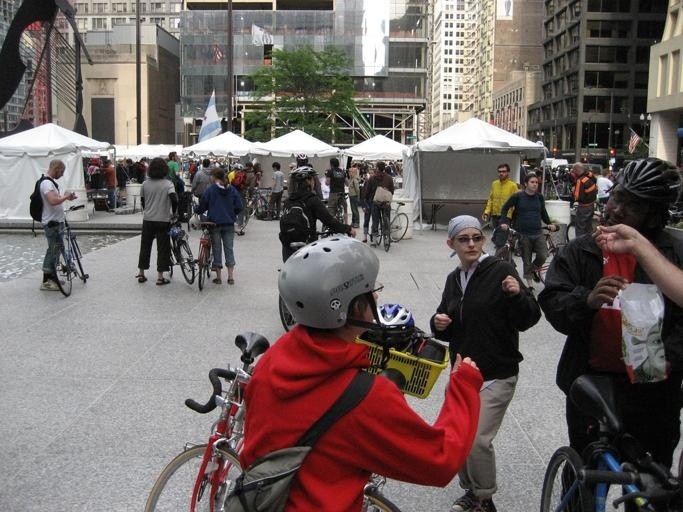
[535,131,544,141]
[125,115,140,149]
[638,113,652,158]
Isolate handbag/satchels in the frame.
[373,186,393,208]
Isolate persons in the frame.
[240,235,484,511]
[38,159,77,291]
[590,221,683,309]
[264,153,395,252]
[427,212,541,511]
[192,168,244,286]
[535,156,682,511]
[88,151,263,217]
[279,165,356,265]
[480,159,615,291]
[136,156,177,286]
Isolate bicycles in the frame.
[595,201,606,219]
[373,205,391,253]
[278,226,353,332]
[492,223,523,265]
[538,370,683,512]
[194,222,216,292]
[321,192,352,224]
[537,224,565,287]
[140,330,272,512]
[166,216,196,285]
[184,184,199,231]
[390,201,409,243]
[361,368,407,511]
[254,185,289,220]
[565,211,603,243]
[50,205,89,298]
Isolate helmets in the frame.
[277,236,379,331]
[615,157,682,208]
[370,305,415,346]
[290,167,320,181]
[234,163,245,170]
[296,154,308,163]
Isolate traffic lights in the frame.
[609,148,617,157]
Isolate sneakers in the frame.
[531,271,540,283]
[41,279,63,290]
[529,287,539,302]
[454,493,495,512]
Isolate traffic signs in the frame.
[588,144,598,147]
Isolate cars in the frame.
[531,156,604,179]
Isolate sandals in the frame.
[212,278,222,284]
[156,277,172,286]
[228,279,235,285]
[138,275,147,282]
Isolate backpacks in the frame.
[278,193,316,245]
[231,170,246,190]
[221,446,312,510]
[30,176,60,222]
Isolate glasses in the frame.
[450,237,483,244]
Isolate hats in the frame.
[447,215,484,242]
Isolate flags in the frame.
[626,131,639,154]
[196,90,221,143]
